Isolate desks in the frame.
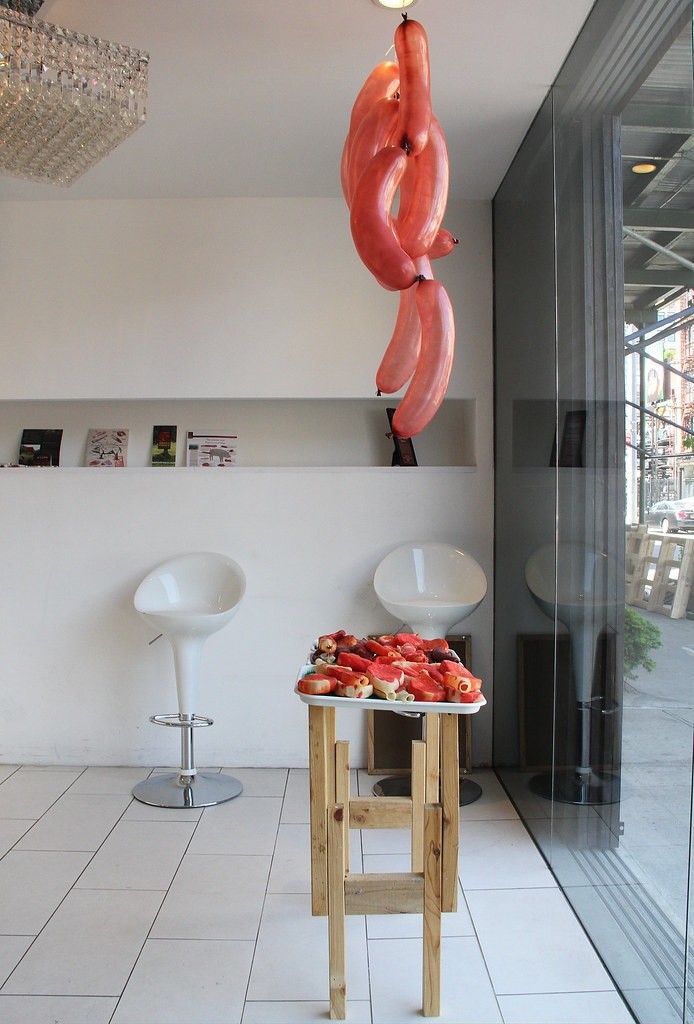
[297,642,487,1021]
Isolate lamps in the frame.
[0,0,151,189]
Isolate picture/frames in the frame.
[515,633,618,774]
[562,409,587,468]
[386,407,419,466]
[366,634,474,777]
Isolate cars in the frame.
[644,501,694,535]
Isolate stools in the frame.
[523,541,638,808]
[371,541,489,809]
[131,551,246,808]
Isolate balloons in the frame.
[339,10,458,444]
